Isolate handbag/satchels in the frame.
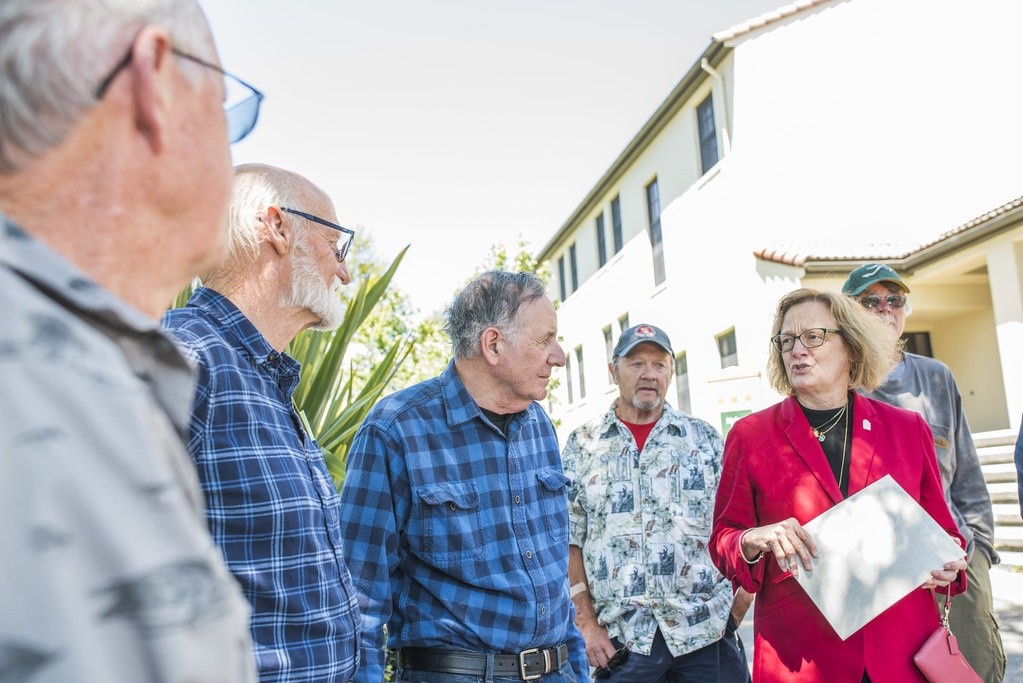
[913,583,986,683]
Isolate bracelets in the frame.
[738,527,765,564]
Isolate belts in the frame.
[396,643,569,681]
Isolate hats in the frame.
[842,263,910,297]
[613,324,674,360]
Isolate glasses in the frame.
[860,295,907,308]
[94,35,264,147]
[591,647,629,679]
[771,325,842,352]
[259,207,356,264]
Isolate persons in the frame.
[340,271,591,683]
[840,263,1007,683]
[706,288,968,683]
[562,323,753,683]
[160,163,359,683]
[1,1,265,683]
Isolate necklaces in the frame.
[813,402,848,488]
